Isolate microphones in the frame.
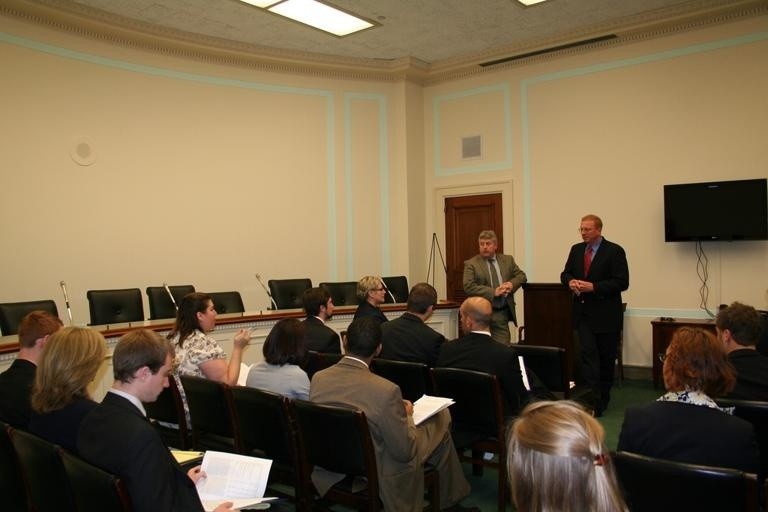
[59,281,73,324]
[163,280,180,319]
[256,274,278,310]
[375,273,397,303]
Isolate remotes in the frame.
[661,317,674,321]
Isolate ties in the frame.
[487,259,505,308]
[583,245,592,278]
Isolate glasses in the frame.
[371,288,384,292]
[656,352,668,362]
[580,228,591,231]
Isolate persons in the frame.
[247,318,312,400]
[615,326,762,475]
[75,327,241,512]
[166,295,254,387]
[300,275,525,413]
[462,230,527,345]
[504,398,629,512]
[715,301,768,402]
[309,317,471,511]
[559,214,630,418]
[0,310,64,433]
[22,326,110,466]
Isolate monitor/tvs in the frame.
[664,178,767,243]
